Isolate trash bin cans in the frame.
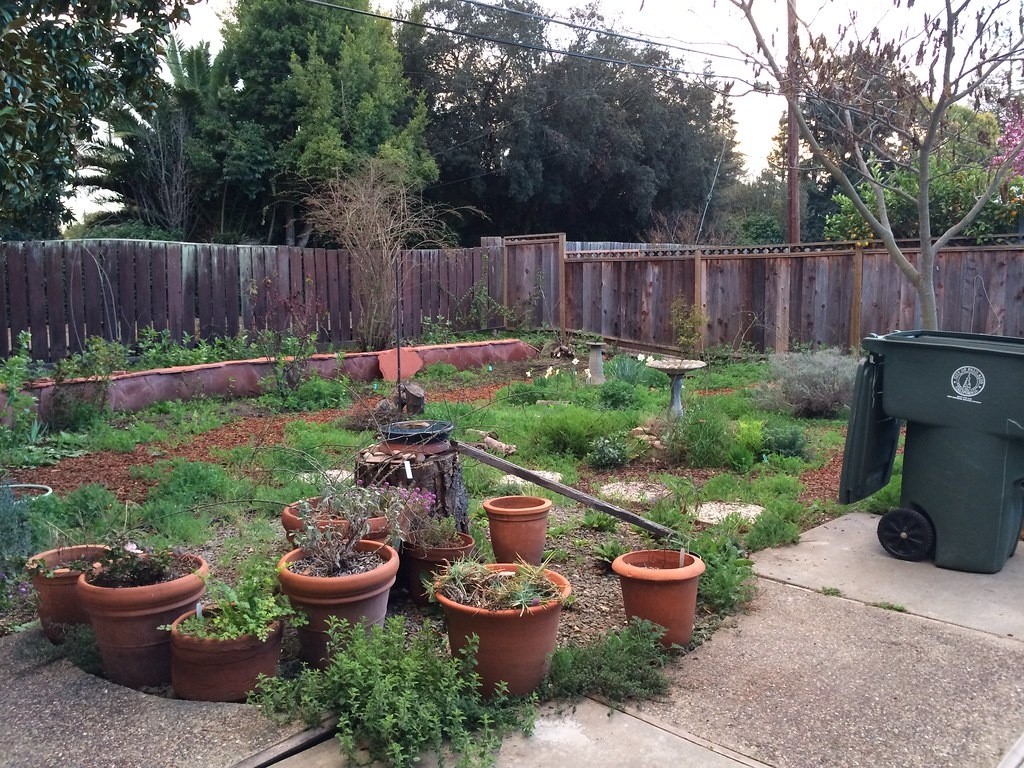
[838,329,1024,576]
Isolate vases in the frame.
[0,419,708,702]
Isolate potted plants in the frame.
[162,552,309,704]
